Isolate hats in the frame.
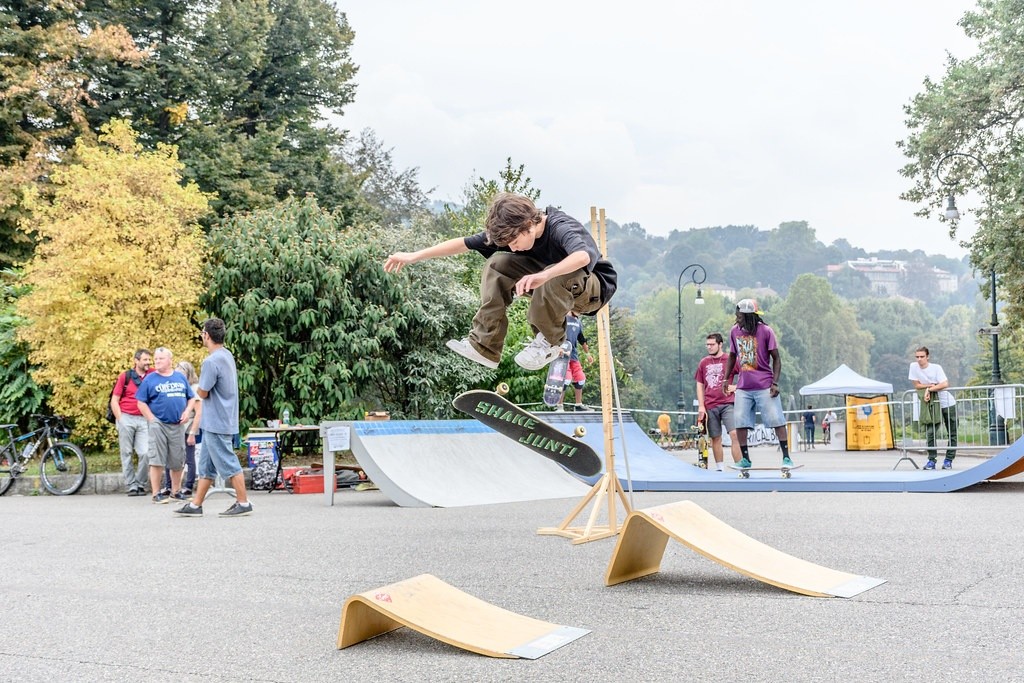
[737,298,765,315]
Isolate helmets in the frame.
[53,427,72,439]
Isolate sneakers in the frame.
[173,502,203,516]
[513,330,562,370]
[782,456,794,469]
[446,339,500,370]
[573,404,595,412]
[923,460,935,469]
[152,494,169,503]
[941,458,952,469]
[168,491,188,502]
[730,458,751,469]
[217,501,253,517]
[557,404,565,412]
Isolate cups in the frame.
[273,420,279,428]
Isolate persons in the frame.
[694,332,742,471]
[384,193,618,370]
[824,411,837,440]
[558,310,595,411]
[174,318,253,517]
[722,299,793,468]
[908,347,959,469]
[135,347,195,504]
[801,405,816,449]
[161,361,202,498]
[110,348,156,495]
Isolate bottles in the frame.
[23,442,34,457]
[283,409,289,424]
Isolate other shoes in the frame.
[181,488,192,497]
[128,489,138,496]
[138,487,146,496]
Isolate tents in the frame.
[800,364,896,442]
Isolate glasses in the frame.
[705,343,718,347]
[200,331,208,337]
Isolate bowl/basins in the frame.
[280,424,288,429]
[267,420,273,427]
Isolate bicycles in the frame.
[0,413,87,497]
[822,421,830,446]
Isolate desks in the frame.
[647,431,699,452]
[249,423,320,495]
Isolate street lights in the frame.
[677,263,707,440]
[936,153,1010,447]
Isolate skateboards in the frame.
[542,341,572,407]
[691,412,708,469]
[451,382,603,478]
[726,464,804,479]
